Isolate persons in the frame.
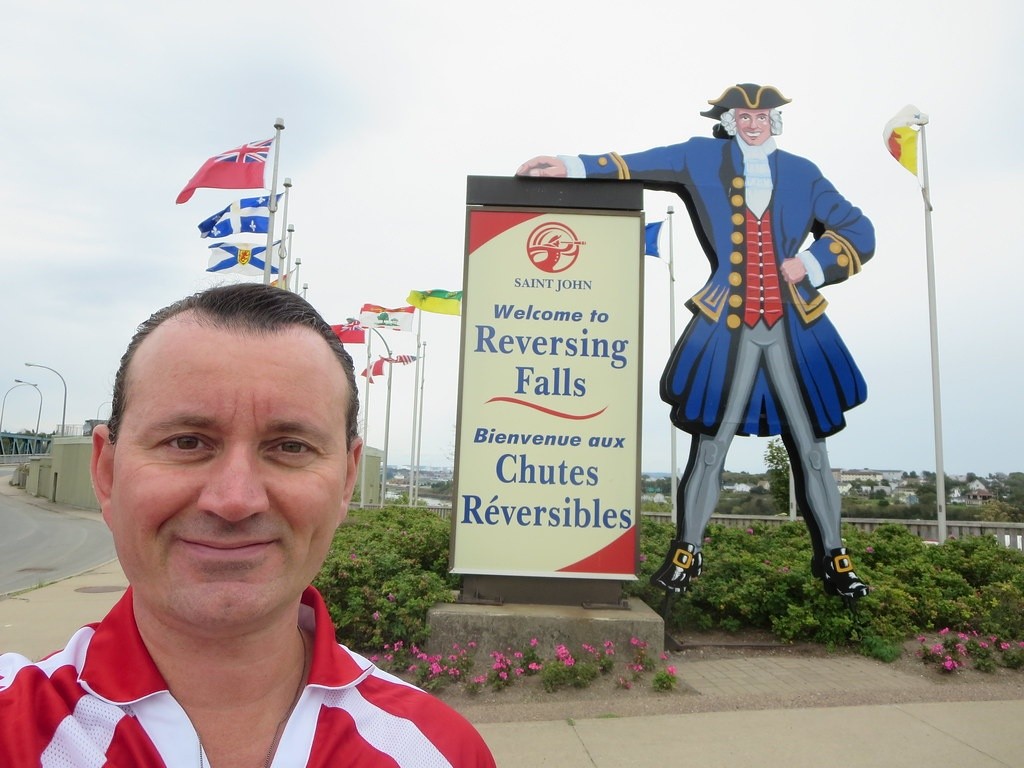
[0,282,496,768]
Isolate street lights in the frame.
[0,384,38,433]
[26,363,67,437]
[347,318,391,508]
[16,380,42,436]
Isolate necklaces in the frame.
[199,622,306,768]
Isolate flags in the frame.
[207,240,282,276]
[362,358,384,384]
[271,270,295,289]
[360,304,413,332]
[176,137,273,204]
[379,355,423,365]
[329,322,364,344]
[198,192,284,239]
[882,106,927,174]
[406,289,463,316]
